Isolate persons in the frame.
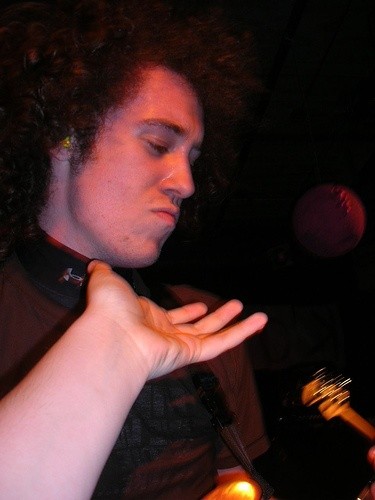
[0,0,274,500]
[0,259,269,500]
[357,446,375,500]
